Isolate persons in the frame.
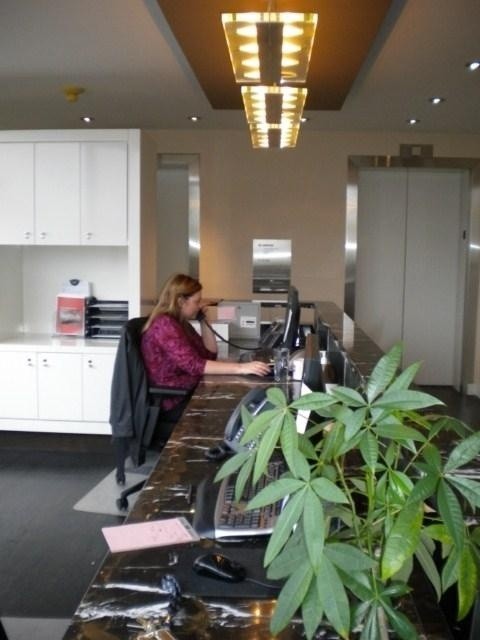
[137,271,273,419]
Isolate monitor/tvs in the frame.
[281,285,301,351]
[297,332,326,470]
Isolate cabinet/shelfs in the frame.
[1,130,142,436]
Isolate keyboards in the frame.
[190,459,286,541]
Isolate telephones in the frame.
[196,310,206,321]
[224,385,288,455]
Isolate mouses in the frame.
[194,550,246,587]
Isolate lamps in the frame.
[221,0,318,149]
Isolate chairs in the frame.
[108,316,190,512]
